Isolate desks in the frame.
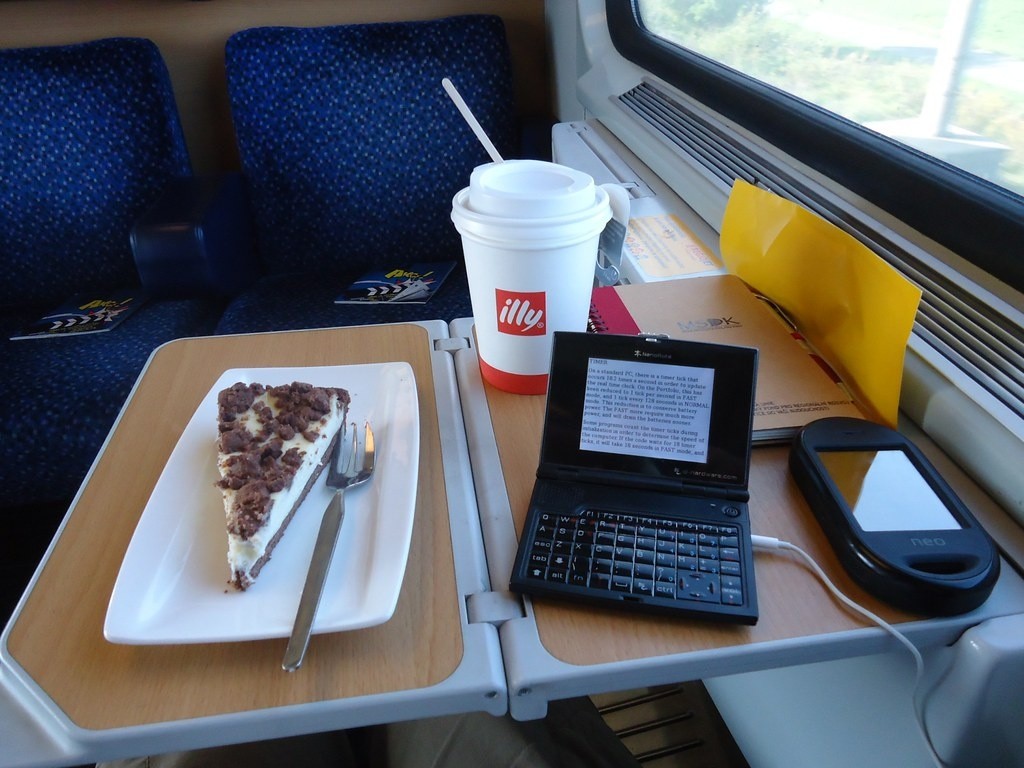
[0,275,1023,768]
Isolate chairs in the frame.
[207,11,553,339]
[0,35,212,536]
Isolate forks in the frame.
[281,420,377,673]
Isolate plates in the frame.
[102,361,421,647]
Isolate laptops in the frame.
[508,331,759,625]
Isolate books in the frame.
[587,275,880,448]
[7,291,156,341]
[333,259,458,305]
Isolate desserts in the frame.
[215,381,351,590]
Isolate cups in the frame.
[450,158,615,396]
